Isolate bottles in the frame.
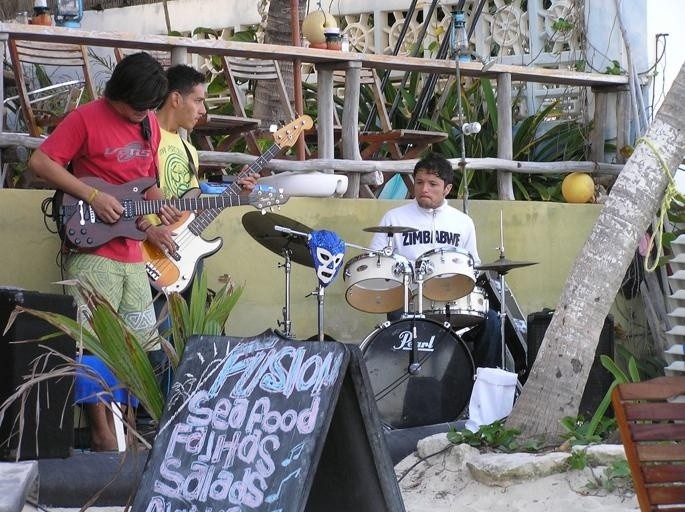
[15,11,29,25]
[340,35,349,52]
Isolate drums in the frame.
[412,285,489,326]
[359,314,475,430]
[343,252,416,313]
[416,245,477,301]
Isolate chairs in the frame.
[611,376,685,512]
[7,33,449,199]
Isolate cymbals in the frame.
[242,211,318,267]
[363,226,419,235]
[473,258,538,274]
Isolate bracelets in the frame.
[143,223,154,232]
[87,187,98,205]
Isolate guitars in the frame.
[141,114,314,298]
[61,176,289,250]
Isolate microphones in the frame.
[408,326,422,375]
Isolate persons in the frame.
[141,62,262,418]
[369,149,503,372]
[28,50,185,454]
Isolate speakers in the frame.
[0,287,78,463]
